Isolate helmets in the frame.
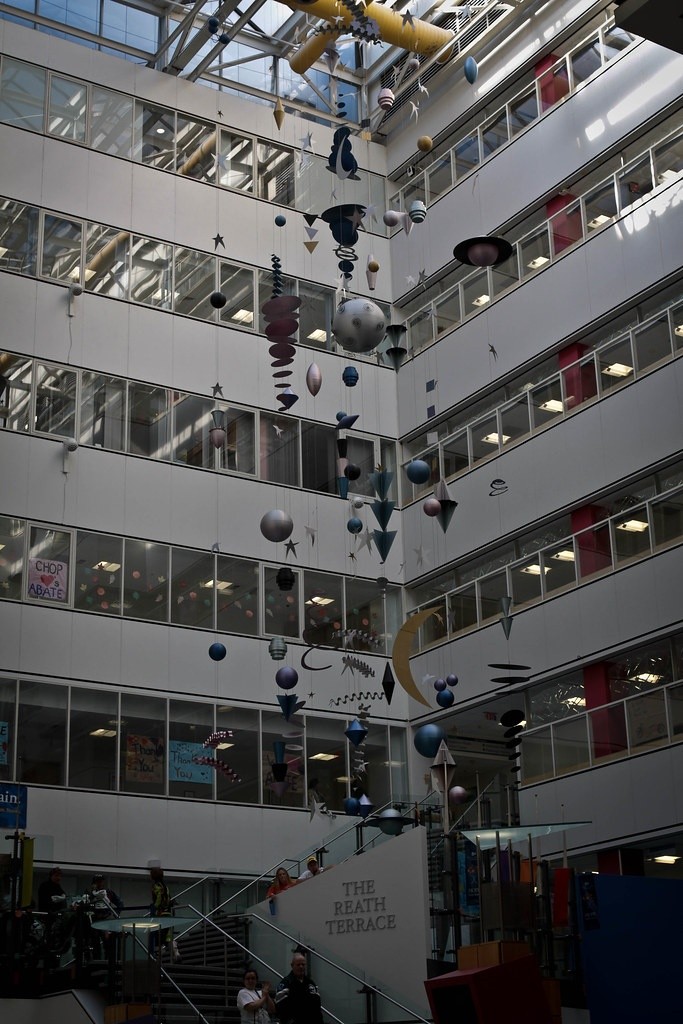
[151,868,163,880]
[88,873,104,889]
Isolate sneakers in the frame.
[174,951,182,962]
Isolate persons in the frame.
[72,873,125,966]
[148,867,182,964]
[39,867,67,919]
[276,953,324,1024]
[299,857,335,881]
[236,969,276,1024]
[266,867,301,898]
[307,778,326,811]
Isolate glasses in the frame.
[245,976,257,980]
[293,961,305,966]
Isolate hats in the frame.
[47,869,60,880]
[307,856,317,868]
